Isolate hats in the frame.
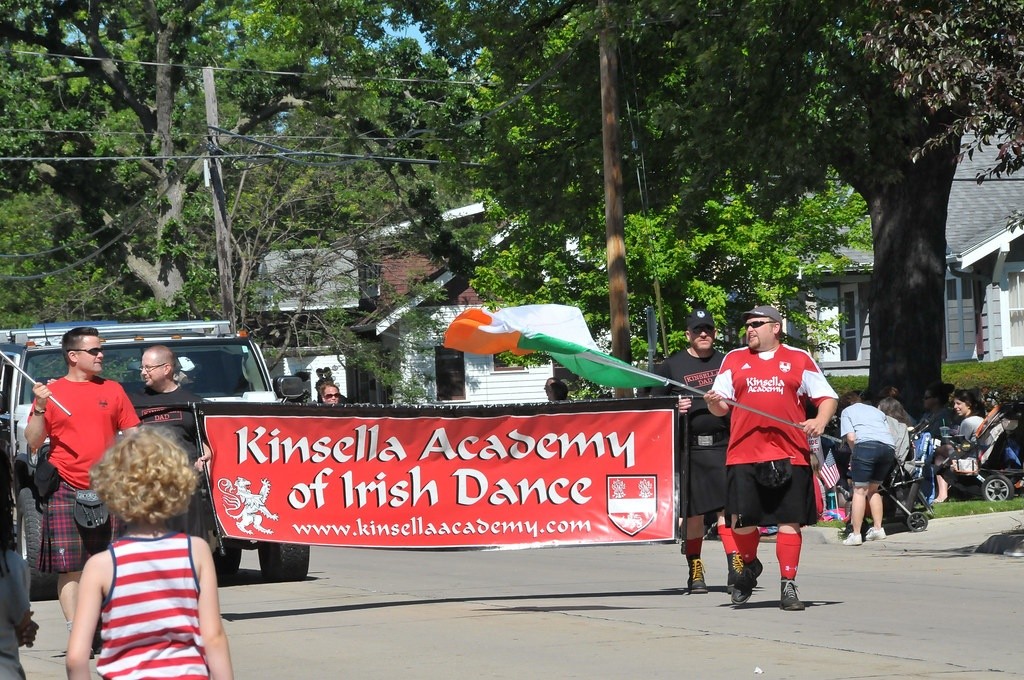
[687,309,715,329]
[742,305,782,325]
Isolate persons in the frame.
[919,383,991,502]
[651,308,758,594]
[0,448,39,680]
[65,426,235,680]
[841,387,917,547]
[544,377,568,401]
[318,381,339,404]
[701,305,839,611]
[24,327,141,659]
[127,345,212,540]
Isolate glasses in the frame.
[66,347,102,356]
[744,321,776,329]
[323,393,341,399]
[691,325,714,334]
[139,363,168,372]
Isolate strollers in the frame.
[837,418,929,533]
[930,402,1015,503]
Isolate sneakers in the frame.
[726,552,745,594]
[686,554,708,593]
[843,533,863,546]
[865,527,887,541]
[731,558,763,604]
[779,577,806,611]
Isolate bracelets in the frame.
[34,406,46,414]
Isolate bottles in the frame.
[827,492,835,510]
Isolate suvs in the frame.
[0,317,318,604]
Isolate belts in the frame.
[689,431,728,446]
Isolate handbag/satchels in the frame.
[33,458,59,496]
[753,458,792,488]
[74,490,109,529]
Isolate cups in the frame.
[939,427,950,436]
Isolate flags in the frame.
[820,449,840,489]
[443,304,666,387]
